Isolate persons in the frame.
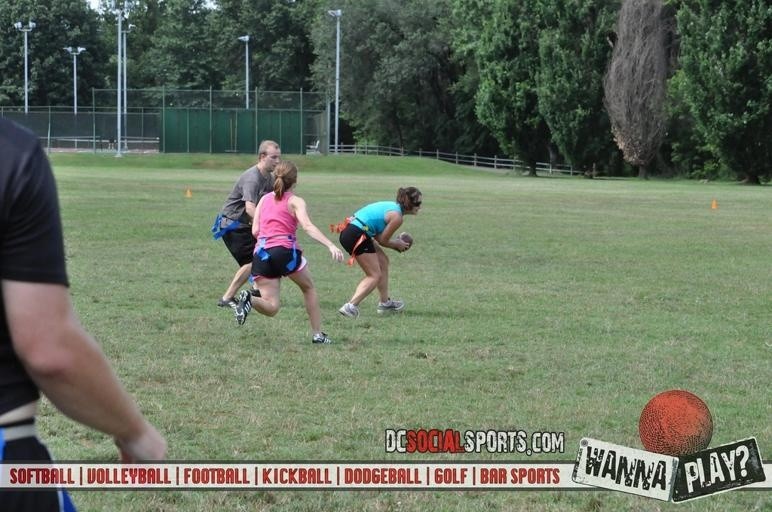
[211,139,282,309]
[339,187,422,318]
[1,114,168,512]
[236,160,344,344]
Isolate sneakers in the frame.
[218,288,260,325]
[312,332,333,344]
[338,303,359,318]
[377,299,404,313]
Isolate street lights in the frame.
[62,46,86,116]
[110,7,137,158]
[13,20,36,114]
[327,8,343,155]
[237,35,252,111]
